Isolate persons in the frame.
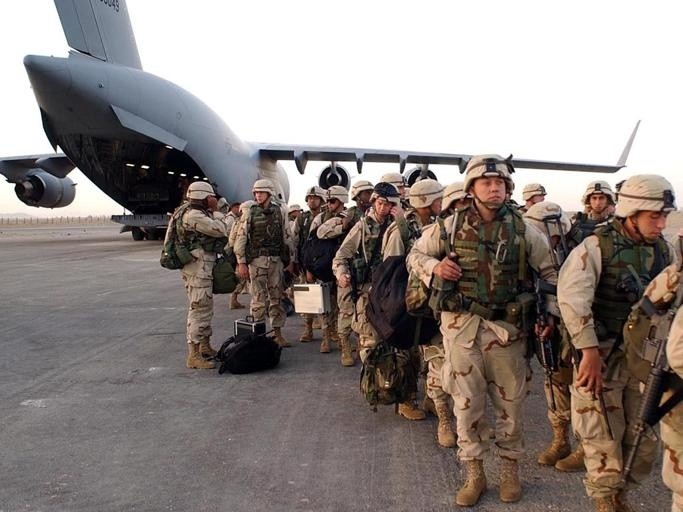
[214,169,468,448]
[523,173,682,511]
[405,153,565,507]
[175,181,230,369]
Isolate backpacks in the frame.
[623,263,683,386]
[159,218,192,270]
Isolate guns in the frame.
[531,268,555,411]
[542,214,612,441]
[620,235,681,484]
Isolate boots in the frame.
[537,423,571,466]
[435,401,457,448]
[186,336,218,369]
[320,324,332,353]
[555,442,586,474]
[228,292,245,310]
[299,317,314,342]
[274,327,292,348]
[340,337,355,366]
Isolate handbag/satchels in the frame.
[365,254,440,350]
[302,228,347,277]
[359,339,417,407]
[219,336,282,374]
[212,260,238,295]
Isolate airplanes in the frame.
[0,0,643,244]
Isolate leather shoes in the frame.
[455,457,488,506]
[499,455,523,503]
[398,397,437,420]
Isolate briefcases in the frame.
[292,281,332,316]
[233,314,265,337]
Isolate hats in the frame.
[252,178,277,196]
[216,197,257,212]
[581,180,616,203]
[408,178,465,211]
[350,172,407,202]
[288,203,303,213]
[186,181,216,199]
[305,185,349,204]
[616,173,677,218]
[522,183,572,238]
[462,154,517,190]
